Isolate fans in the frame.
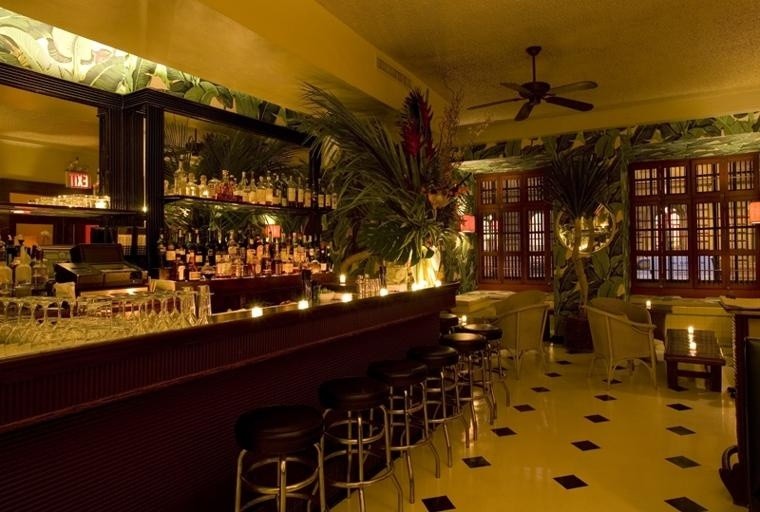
[466,43,602,122]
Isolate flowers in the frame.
[299,74,481,293]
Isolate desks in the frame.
[663,327,727,392]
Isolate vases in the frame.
[409,245,441,289]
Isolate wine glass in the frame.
[0,289,215,352]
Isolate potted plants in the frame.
[540,147,623,354]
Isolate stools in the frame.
[367,360,441,503]
[228,403,329,511]
[440,313,459,334]
[439,333,497,426]
[404,344,471,468]
[317,376,406,511]
[461,324,510,420]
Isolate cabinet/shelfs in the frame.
[150,105,318,277]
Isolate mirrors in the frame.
[0,79,121,211]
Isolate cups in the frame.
[311,280,321,300]
[354,278,381,293]
[35,194,113,209]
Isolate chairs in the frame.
[485,288,659,391]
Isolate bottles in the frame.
[155,225,333,281]
[0,235,49,295]
[301,269,311,299]
[164,153,340,211]
